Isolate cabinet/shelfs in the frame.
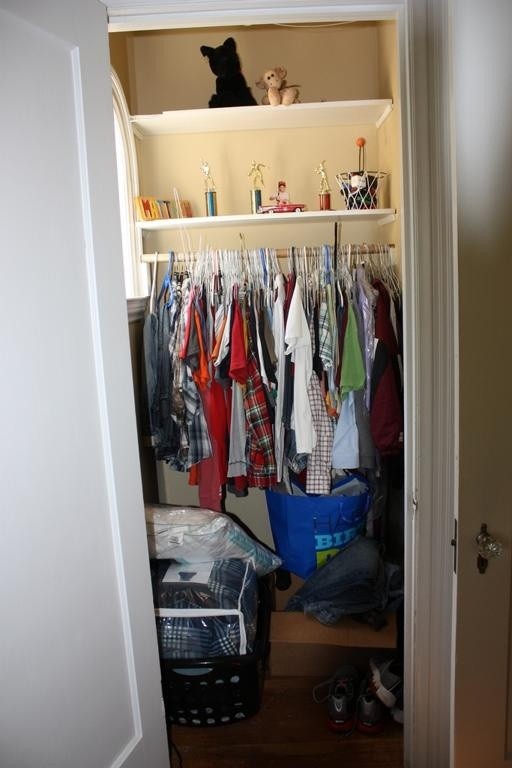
[130,97,396,230]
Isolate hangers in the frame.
[146,241,401,313]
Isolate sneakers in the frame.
[358,667,388,735]
[315,666,356,733]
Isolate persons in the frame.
[247,160,268,190]
[199,156,217,192]
[315,161,331,193]
[269,181,289,204]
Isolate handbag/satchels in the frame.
[265,470,372,581]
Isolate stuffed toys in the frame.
[250,65,302,106]
[199,34,259,107]
